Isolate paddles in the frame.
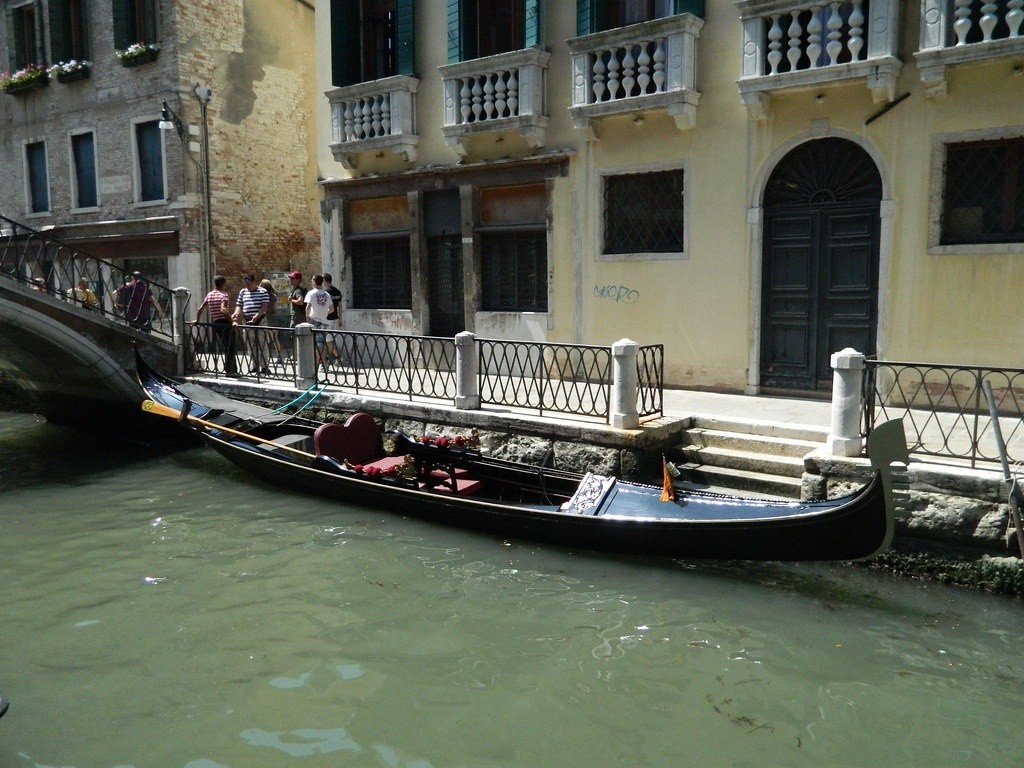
[141,399,317,459]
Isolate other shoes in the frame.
[322,357,330,366]
[249,367,260,374]
[225,372,242,379]
[285,355,297,361]
[334,356,344,366]
[259,367,271,375]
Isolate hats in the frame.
[288,272,302,281]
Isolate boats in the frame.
[131,339,911,564]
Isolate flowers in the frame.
[116,39,157,58]
[0,63,45,90]
[46,58,87,79]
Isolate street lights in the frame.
[159,83,213,341]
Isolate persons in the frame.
[191,275,243,377]
[259,279,283,364]
[34,278,46,293]
[69,277,96,310]
[112,271,164,334]
[285,272,344,366]
[230,274,271,373]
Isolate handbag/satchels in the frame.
[244,314,266,326]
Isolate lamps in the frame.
[158,98,184,140]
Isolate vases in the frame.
[57,67,89,84]
[120,49,159,69]
[3,76,49,95]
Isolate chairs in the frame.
[313,413,483,497]
